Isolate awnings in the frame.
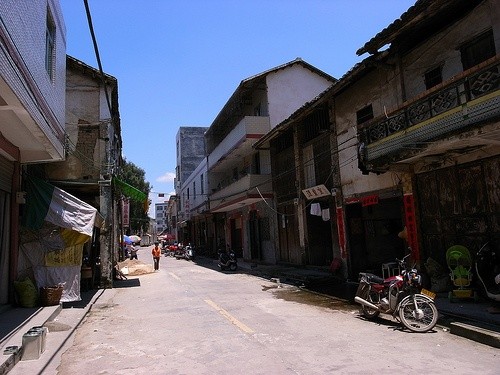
[115,175,147,206]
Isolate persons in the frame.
[150,242,162,273]
[124,242,128,258]
[130,242,140,261]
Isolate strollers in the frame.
[446,245,484,304]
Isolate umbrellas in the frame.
[129,235,142,243]
[120,234,133,243]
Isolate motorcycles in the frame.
[217,248,239,271]
[161,241,195,261]
[354,247,439,333]
